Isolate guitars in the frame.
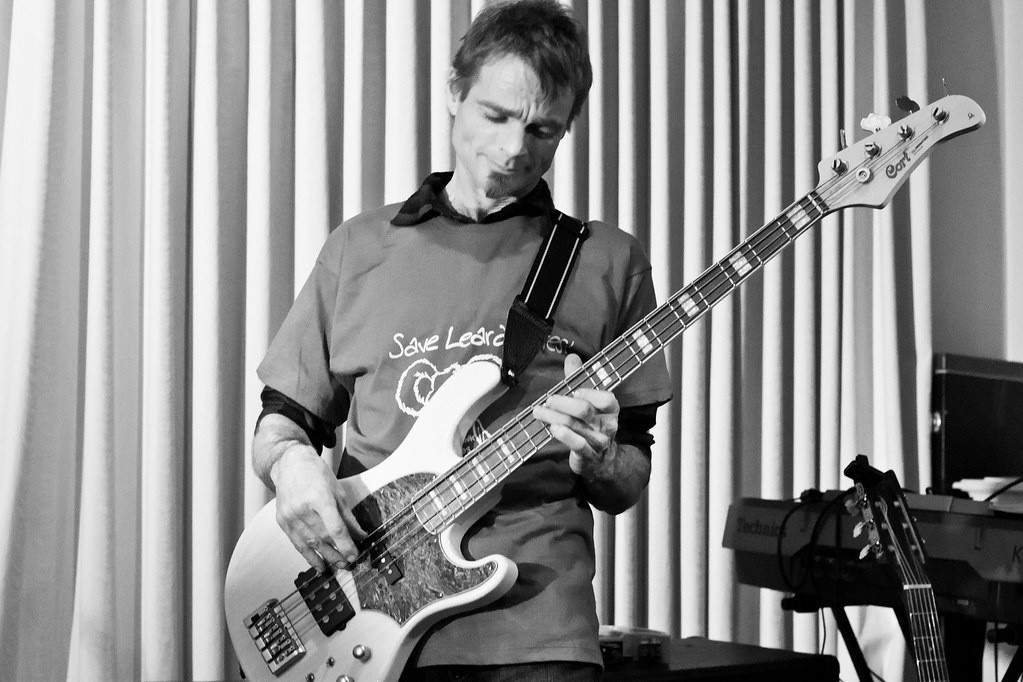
[843,453,951,682]
[223,76,988,682]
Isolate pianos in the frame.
[721,488,1023,615]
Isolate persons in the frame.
[250,0,676,682]
